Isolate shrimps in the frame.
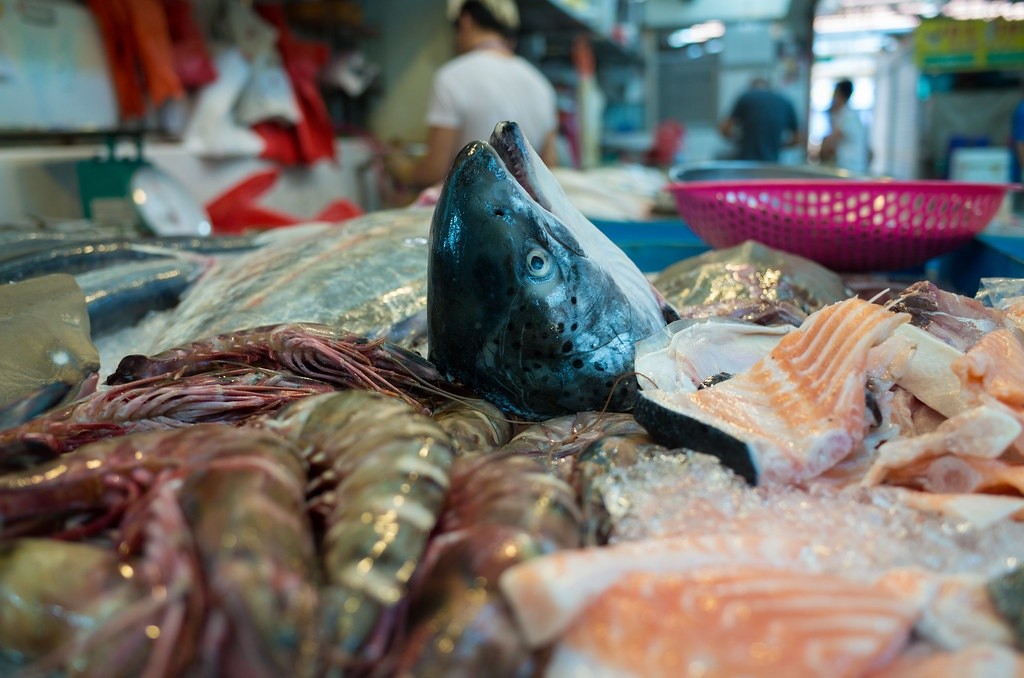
[0,321,651,678]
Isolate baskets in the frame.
[664,180,1022,269]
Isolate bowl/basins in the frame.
[667,161,975,236]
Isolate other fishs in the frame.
[0,117,1024,506]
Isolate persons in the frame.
[716,68,878,176]
[385,1,559,191]
